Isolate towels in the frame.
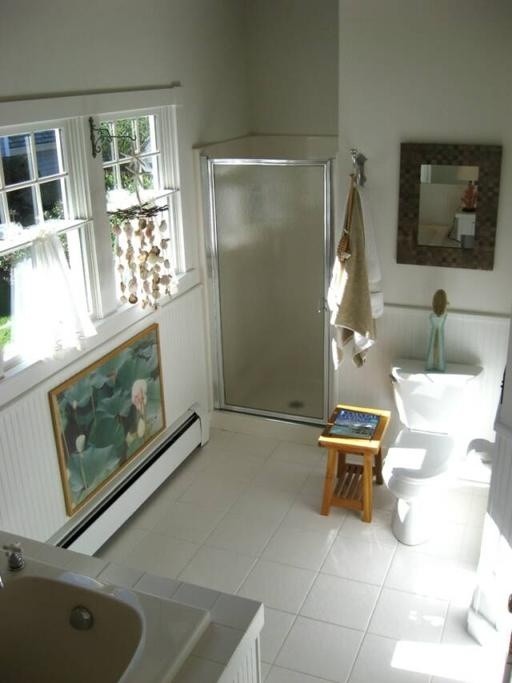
[327,183,376,372]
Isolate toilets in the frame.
[382,356,484,548]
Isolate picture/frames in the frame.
[45,322,167,518]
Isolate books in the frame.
[327,408,382,441]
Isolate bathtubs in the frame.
[0,547,218,682]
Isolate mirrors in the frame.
[395,141,504,271]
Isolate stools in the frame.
[318,402,391,523]
